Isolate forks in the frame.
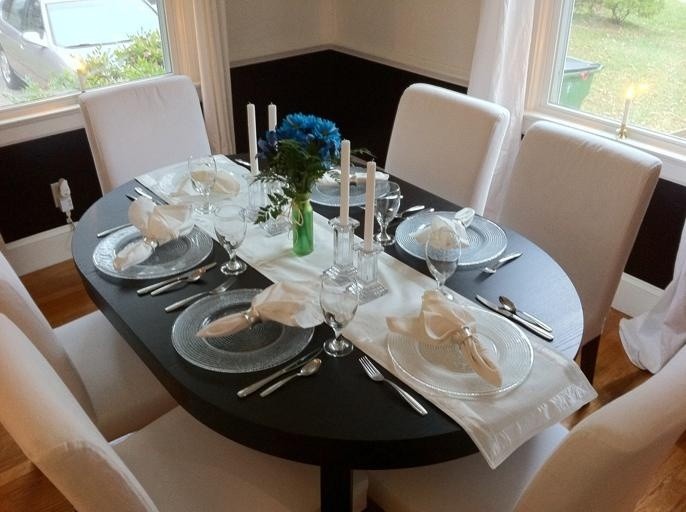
[161,275,237,314]
[478,254,521,274]
[356,354,429,417]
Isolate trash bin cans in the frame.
[559,56,603,111]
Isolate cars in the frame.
[0,1,158,89]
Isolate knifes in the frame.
[135,256,220,295]
[474,289,553,344]
[234,343,327,400]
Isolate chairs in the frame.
[0,249,226,443]
[0,315,371,512]
[76,73,225,192]
[493,119,663,402]
[382,78,512,217]
[358,347,686,512]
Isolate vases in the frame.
[286,196,317,256]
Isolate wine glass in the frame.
[208,204,250,276]
[315,273,360,358]
[371,179,399,247]
[185,152,218,219]
[424,230,460,301]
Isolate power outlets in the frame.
[50,178,75,215]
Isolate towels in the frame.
[407,205,477,245]
[387,288,503,389]
[108,193,196,277]
[193,274,325,342]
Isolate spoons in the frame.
[496,294,552,333]
[256,359,326,400]
[150,272,206,296]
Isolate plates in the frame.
[386,302,534,400]
[396,212,504,264]
[302,165,389,209]
[162,170,241,201]
[91,220,217,280]
[168,286,312,372]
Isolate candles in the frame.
[339,140,350,225]
[247,103,258,175]
[364,158,376,254]
[268,104,277,174]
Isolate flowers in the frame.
[253,114,344,196]
